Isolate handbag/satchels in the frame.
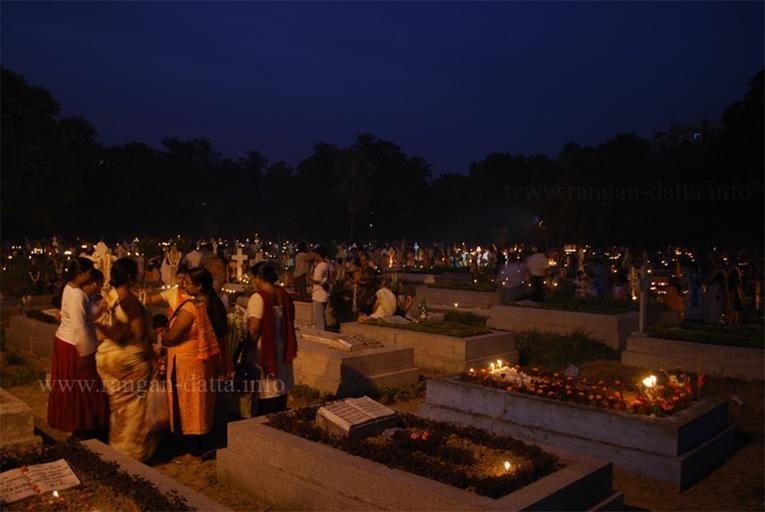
[92,296,138,344]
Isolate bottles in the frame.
[419,299,428,319]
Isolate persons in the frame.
[1,234,375,468]
[363,234,765,327]
